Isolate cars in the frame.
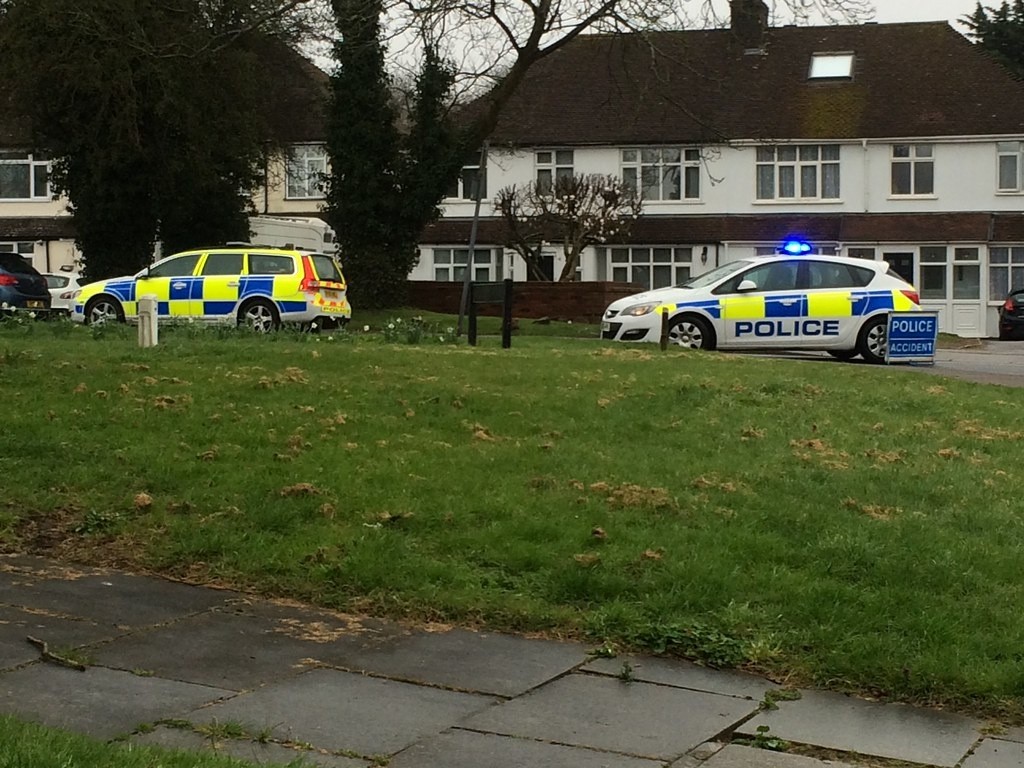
[0,253,53,318]
[997,290,1024,336]
[71,241,352,336]
[601,243,927,362]
[39,272,91,315]
